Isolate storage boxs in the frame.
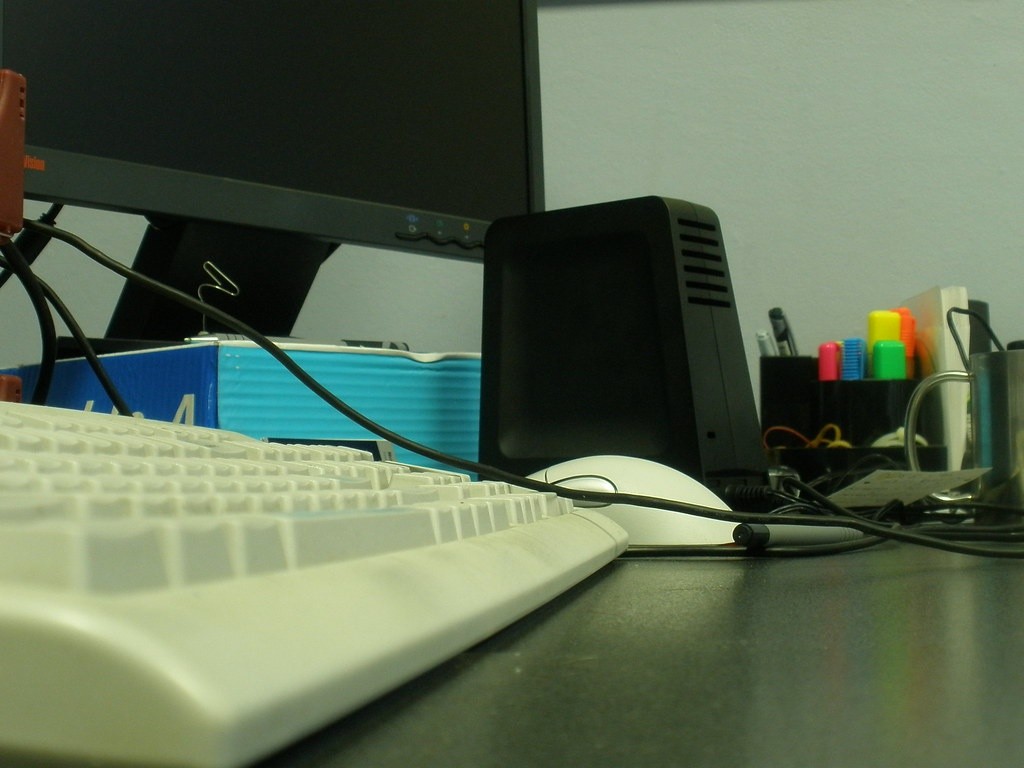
[0,340,481,482]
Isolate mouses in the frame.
[522,455,742,544]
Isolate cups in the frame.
[866,311,901,379]
[904,350,1024,525]
[818,343,841,380]
[872,340,905,381]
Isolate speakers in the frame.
[479,196,777,521]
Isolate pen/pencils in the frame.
[732,522,865,549]
[755,306,798,357]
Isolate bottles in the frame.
[892,308,916,380]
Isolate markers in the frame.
[816,307,917,381]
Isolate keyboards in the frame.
[0,400,628,767]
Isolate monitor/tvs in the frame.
[0,0,546,344]
